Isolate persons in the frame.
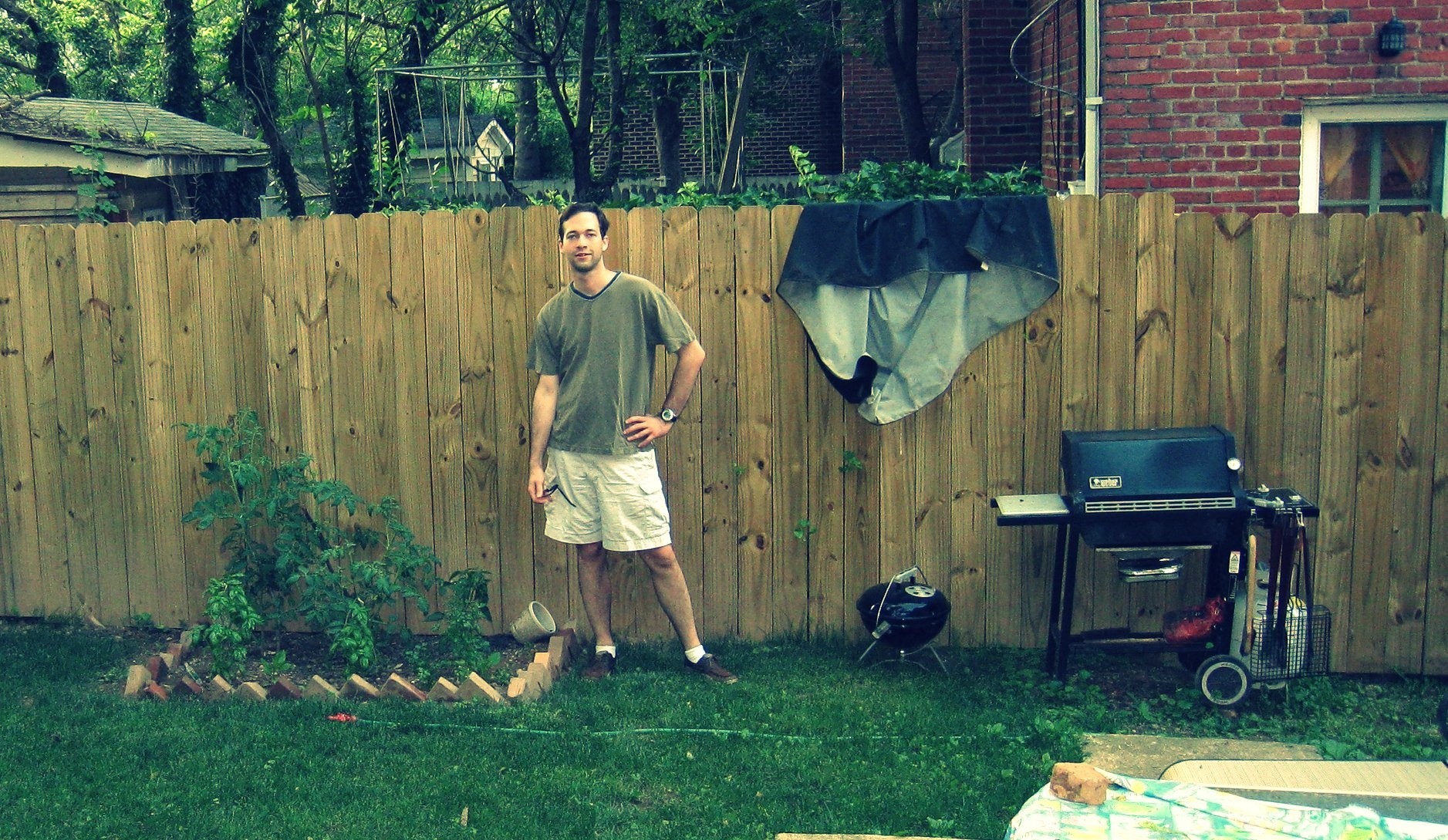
[529,203,741,684]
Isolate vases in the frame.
[509,600,555,646]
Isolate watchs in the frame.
[660,408,677,423]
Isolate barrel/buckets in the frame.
[511,600,557,645]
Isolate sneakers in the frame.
[583,650,617,679]
[685,653,738,684]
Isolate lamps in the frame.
[1379,16,1407,55]
